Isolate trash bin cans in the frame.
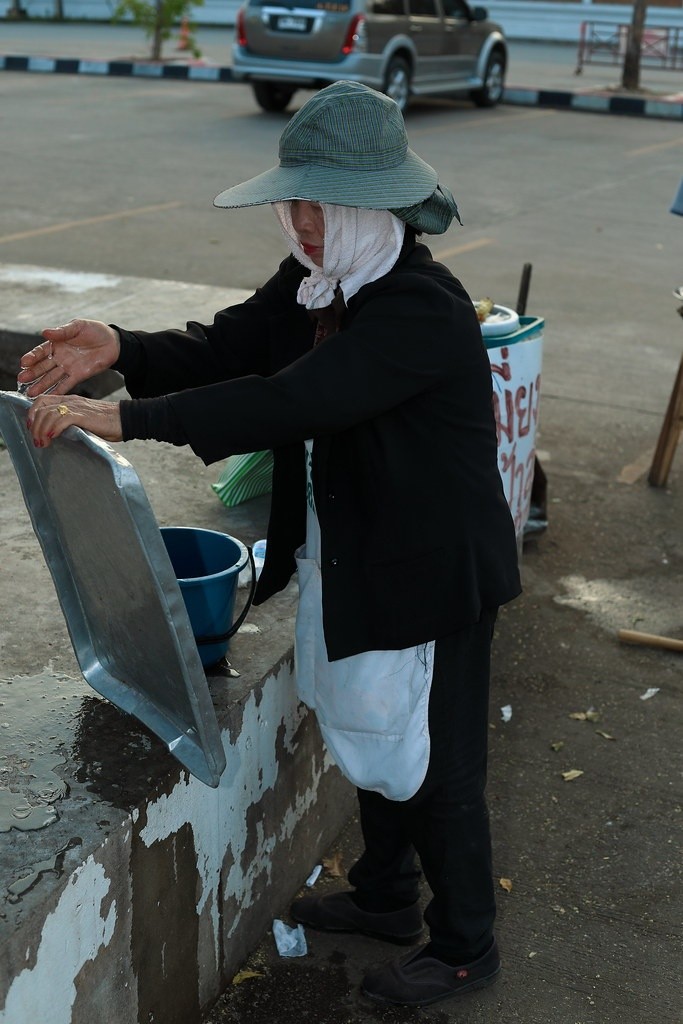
[483,316,547,559]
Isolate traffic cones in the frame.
[176,15,192,51]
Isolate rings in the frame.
[57,405,68,417]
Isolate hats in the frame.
[213,80,464,235]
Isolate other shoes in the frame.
[360,932,502,1008]
[288,890,425,945]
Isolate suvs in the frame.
[228,0,511,117]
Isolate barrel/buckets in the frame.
[160,526,258,669]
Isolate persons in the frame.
[670,173,683,301]
[19,80,523,1006]
